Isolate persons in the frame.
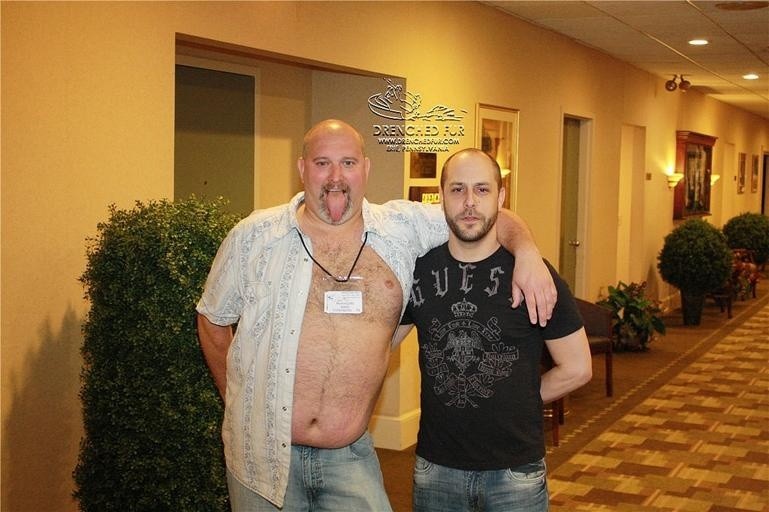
[390,147,594,512]
[193,117,560,512]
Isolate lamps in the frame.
[668,174,684,187]
[679,74,691,90]
[665,76,677,91]
[500,169,511,176]
[710,174,720,185]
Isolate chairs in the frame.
[705,248,760,319]
[559,298,613,425]
[540,350,559,446]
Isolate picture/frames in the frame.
[738,152,745,193]
[751,155,760,193]
[474,102,520,215]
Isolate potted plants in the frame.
[656,218,733,326]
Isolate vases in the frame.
[618,331,642,351]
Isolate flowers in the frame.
[596,280,665,344]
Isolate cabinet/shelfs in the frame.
[673,131,718,220]
